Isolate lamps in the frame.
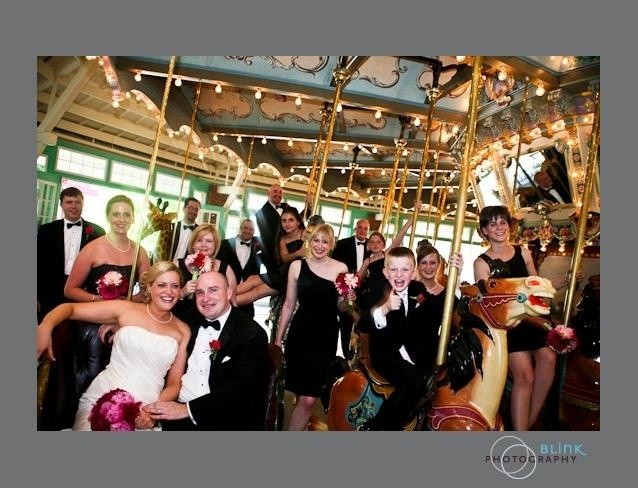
[86,54,551,209]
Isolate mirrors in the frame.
[470,130,579,212]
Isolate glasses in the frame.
[185,205,198,212]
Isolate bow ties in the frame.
[201,320,220,330]
[66,221,81,228]
[275,204,282,209]
[357,242,367,246]
[183,225,193,230]
[240,241,250,247]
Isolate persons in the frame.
[37,172,570,431]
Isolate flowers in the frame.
[95,271,129,300]
[184,252,216,301]
[334,273,361,316]
[84,226,94,240]
[86,388,142,431]
[209,340,221,361]
[410,294,426,309]
[544,322,577,354]
